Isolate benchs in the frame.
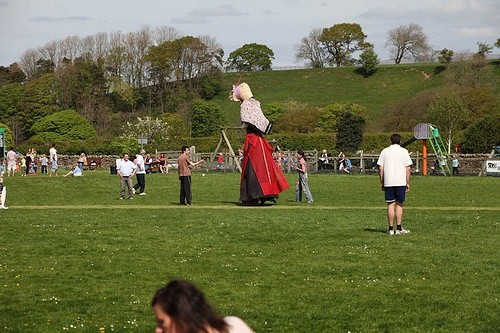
[150,161,166,173]
[82,157,101,171]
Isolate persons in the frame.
[49,144,58,177]
[294,150,313,205]
[432,149,460,176]
[63,164,81,178]
[78,152,87,170]
[152,280,256,333]
[217,153,224,169]
[430,125,439,150]
[240,126,290,206]
[236,143,295,172]
[7,147,16,177]
[321,150,350,174]
[177,146,205,206]
[144,154,152,171]
[377,133,413,235]
[132,149,146,195]
[116,153,137,200]
[156,153,169,174]
[15,148,48,176]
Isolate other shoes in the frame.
[132,188,136,195]
[187,204,193,206]
[129,197,133,199]
[139,192,146,195]
[395,227,410,234]
[120,197,123,199]
[181,204,186,205]
[387,229,394,235]
[0,205,9,209]
